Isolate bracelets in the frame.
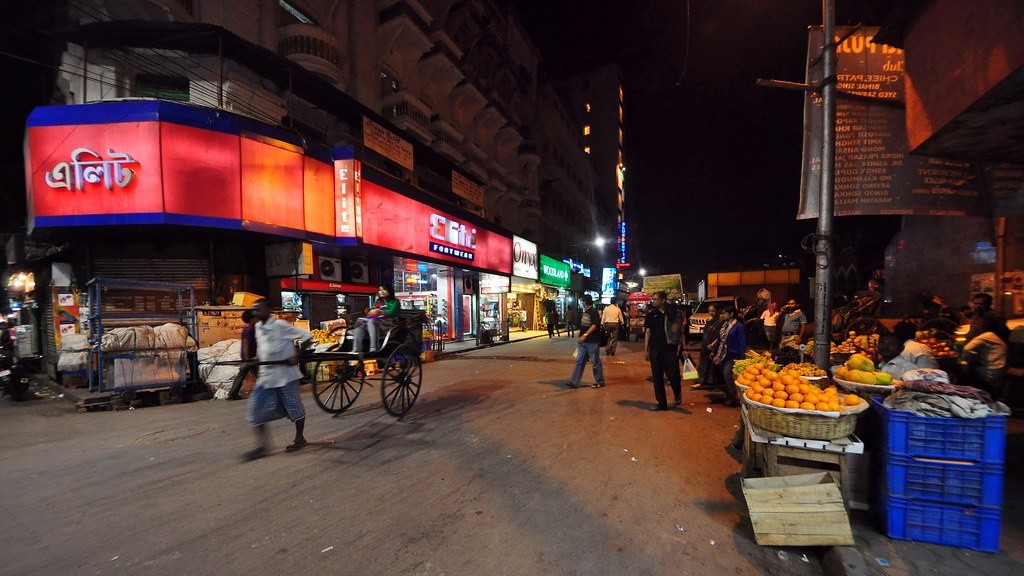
[583,334,587,337]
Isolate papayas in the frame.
[833,354,894,385]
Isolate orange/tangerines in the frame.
[835,330,862,351]
[310,328,337,344]
[737,363,859,411]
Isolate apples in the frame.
[915,330,957,357]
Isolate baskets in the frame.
[798,376,829,391]
[733,380,750,395]
[831,374,897,402]
[742,391,872,440]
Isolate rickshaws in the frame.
[806,290,883,346]
[211,307,429,419]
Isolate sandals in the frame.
[592,383,606,388]
[566,382,578,389]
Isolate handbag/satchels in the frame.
[572,348,590,363]
[599,329,608,348]
[682,358,700,380]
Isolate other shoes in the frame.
[284,439,308,453]
[671,401,680,409]
[240,445,271,461]
[691,383,707,390]
[723,398,738,407]
[225,395,242,401]
[650,404,669,412]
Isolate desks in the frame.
[741,402,863,520]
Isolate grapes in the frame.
[732,355,765,376]
[769,345,814,366]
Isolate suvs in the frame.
[687,295,747,340]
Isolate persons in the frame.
[566,305,574,337]
[710,305,746,404]
[564,294,606,389]
[601,297,625,356]
[352,284,400,352]
[962,311,1008,382]
[545,308,560,339]
[519,308,528,333]
[875,331,919,380]
[776,297,807,349]
[642,291,688,411]
[225,309,260,400]
[690,303,725,390]
[971,293,1006,339]
[893,321,939,369]
[760,302,779,341]
[245,298,313,459]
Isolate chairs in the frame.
[346,310,423,351]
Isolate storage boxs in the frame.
[740,471,854,546]
[420,351,435,363]
[316,361,336,381]
[869,396,1006,554]
[354,359,378,377]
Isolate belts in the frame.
[783,332,799,335]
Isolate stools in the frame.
[431,340,445,350]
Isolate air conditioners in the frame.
[318,256,341,282]
[350,261,369,283]
